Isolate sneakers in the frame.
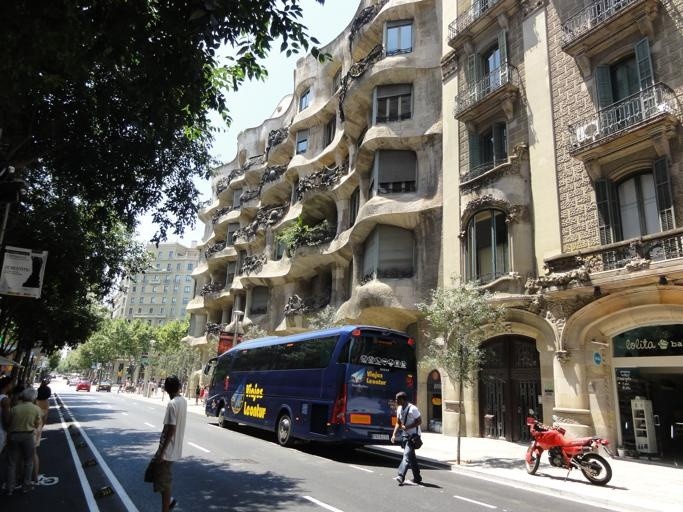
[394,475,422,484]
[1,478,39,496]
[168,496,178,512]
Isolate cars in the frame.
[51,372,112,392]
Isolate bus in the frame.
[203,325,417,450]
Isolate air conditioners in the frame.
[576,119,600,145]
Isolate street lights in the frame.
[233,311,244,347]
[147,340,156,398]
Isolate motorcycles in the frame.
[525,409,612,486]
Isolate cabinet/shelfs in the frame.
[631,397,658,455]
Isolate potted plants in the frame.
[615,444,626,457]
[543,383,553,396]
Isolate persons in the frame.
[143,375,188,512]
[0,375,51,496]
[117,375,209,404]
[390,391,423,484]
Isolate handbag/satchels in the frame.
[407,432,424,450]
